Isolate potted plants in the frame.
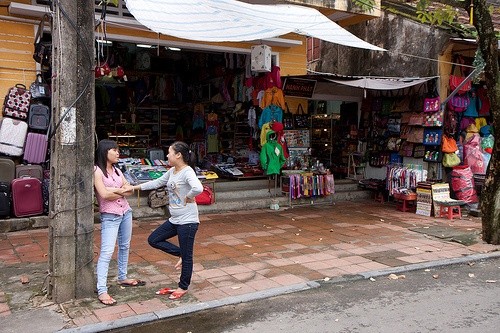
[125,123,140,134]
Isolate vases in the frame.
[131,113,135,123]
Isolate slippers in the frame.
[97,295,117,306]
[157,288,175,295]
[169,290,189,300]
[121,279,146,287]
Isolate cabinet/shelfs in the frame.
[312,113,342,167]
[159,108,179,144]
[96,106,159,144]
[220,107,255,154]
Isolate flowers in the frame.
[127,92,150,112]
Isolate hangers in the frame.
[393,162,423,170]
[209,110,216,137]
[259,41,283,142]
[197,102,201,119]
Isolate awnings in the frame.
[308,69,440,90]
[123,0,388,52]
[449,36,500,58]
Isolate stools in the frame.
[397,199,415,211]
[439,205,461,219]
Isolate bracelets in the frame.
[132,186,134,191]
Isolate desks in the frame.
[109,135,136,143]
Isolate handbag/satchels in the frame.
[282,104,294,130]
[148,186,169,208]
[30,74,49,101]
[34,14,51,66]
[195,186,215,205]
[292,104,310,129]
[442,59,494,168]
[358,84,445,169]
[291,172,335,199]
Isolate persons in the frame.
[93,139,138,306]
[120,141,204,300]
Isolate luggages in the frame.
[0,76,50,219]
